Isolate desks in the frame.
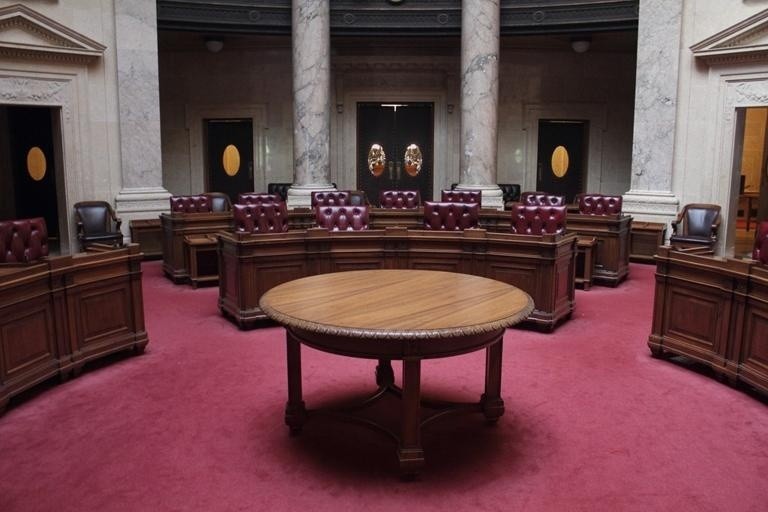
[258,269,536,481]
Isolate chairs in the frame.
[0,217,48,263]
[670,204,721,249]
[752,222,768,264]
[73,201,123,252]
